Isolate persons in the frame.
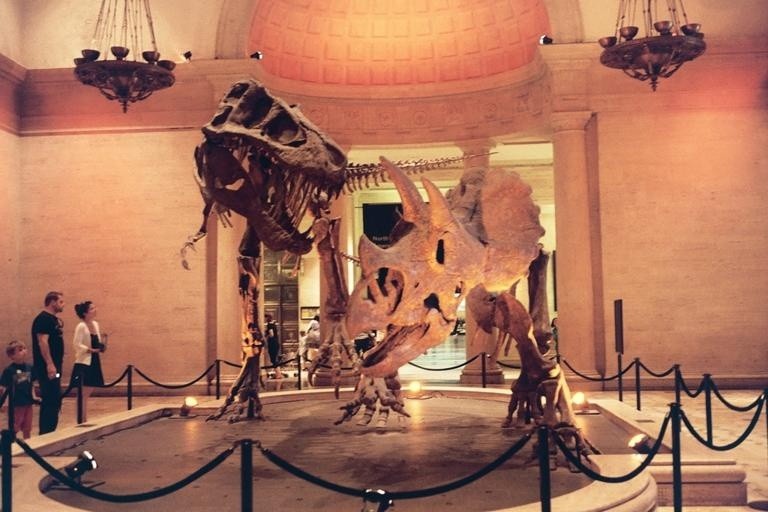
[354,332,370,356]
[551,318,558,353]
[243,313,320,379]
[0,292,104,439]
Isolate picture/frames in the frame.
[300,306,320,320]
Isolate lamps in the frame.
[573,393,599,414]
[74,0,175,113]
[407,381,431,399]
[65,451,105,488]
[599,0,706,91]
[250,52,262,60]
[171,397,197,419]
[360,488,393,512]
[538,35,552,44]
[182,51,192,61]
[628,434,650,454]
